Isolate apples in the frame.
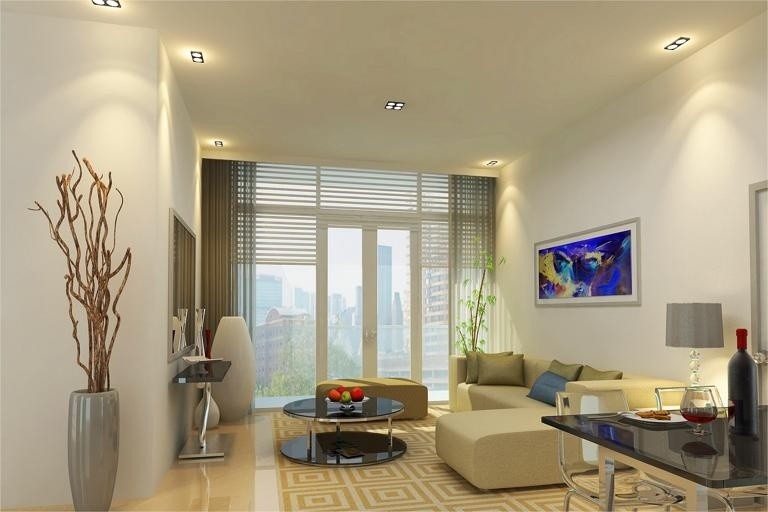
[329,389,341,401]
[337,387,347,394]
[341,391,351,403]
[351,387,365,401]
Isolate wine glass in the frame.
[679,387,718,436]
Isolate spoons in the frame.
[587,412,633,420]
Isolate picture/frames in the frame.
[533,217,640,307]
[167,207,196,362]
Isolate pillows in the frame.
[577,365,622,381]
[527,371,570,407]
[464,351,514,383]
[549,360,583,380]
[477,352,525,385]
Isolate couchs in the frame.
[436,352,687,493]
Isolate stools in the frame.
[315,375,428,420]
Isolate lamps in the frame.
[665,302,724,384]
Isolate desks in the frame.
[173,361,233,460]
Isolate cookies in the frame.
[637,410,671,420]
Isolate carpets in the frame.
[271,404,685,512]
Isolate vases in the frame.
[178,309,189,350]
[68,388,120,510]
[209,316,255,422]
[194,308,220,430]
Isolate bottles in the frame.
[727,328,759,435]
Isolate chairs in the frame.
[653,386,741,511]
[557,390,684,511]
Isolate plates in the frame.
[616,410,689,425]
